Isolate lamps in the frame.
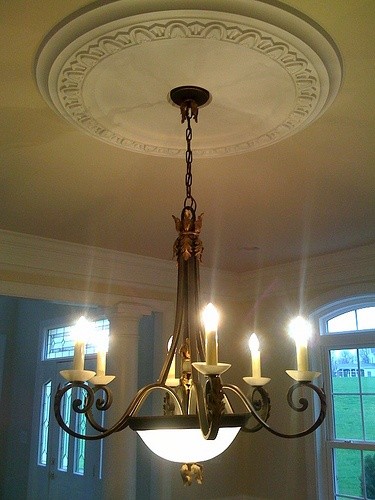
[50,84,329,491]
